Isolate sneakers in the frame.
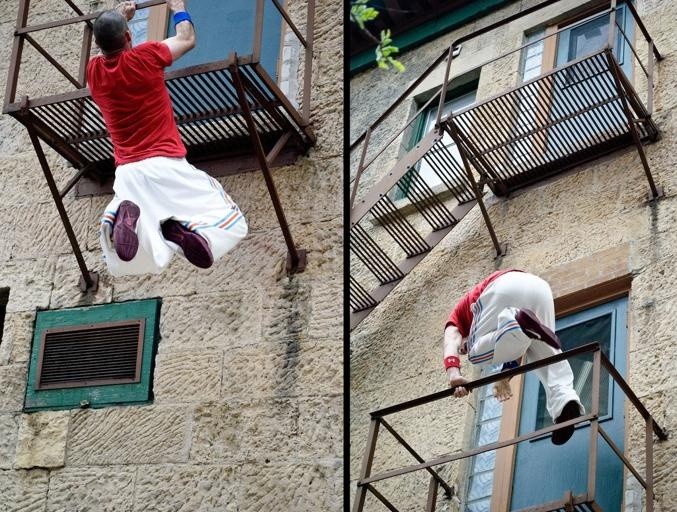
[113,201,141,263]
[517,307,560,348]
[162,218,214,269]
[551,400,578,444]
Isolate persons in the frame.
[441,269,585,445]
[83,0,246,278]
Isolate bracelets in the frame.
[500,360,519,381]
[174,11,195,29]
[443,356,461,371]
